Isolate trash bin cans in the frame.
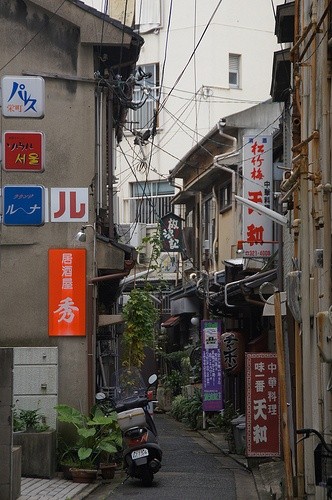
[231,413,247,456]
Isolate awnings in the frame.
[162,315,183,329]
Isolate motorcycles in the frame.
[94,373,162,486]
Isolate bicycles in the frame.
[295,428,332,500]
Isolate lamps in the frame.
[75,224,95,242]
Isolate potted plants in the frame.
[52,404,125,483]
[173,392,236,434]
[12,408,56,477]
